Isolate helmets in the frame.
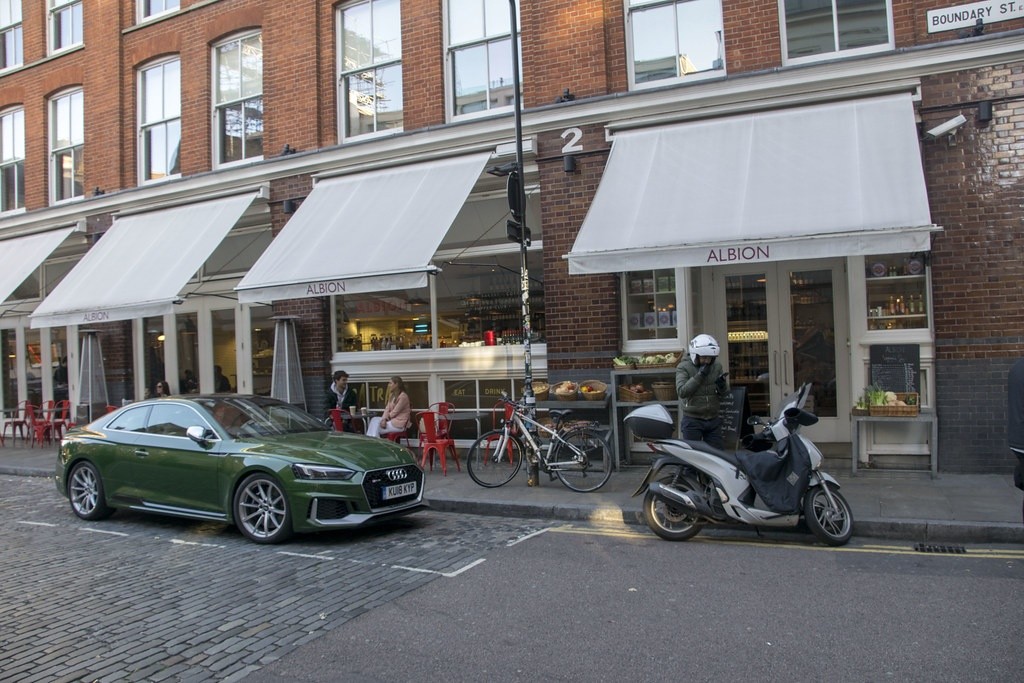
[689,333,720,366]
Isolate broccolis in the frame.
[884,391,915,406]
[639,353,677,364]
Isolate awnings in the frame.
[1,226,76,305]
[233,150,496,305]
[29,189,260,329]
[563,91,944,276]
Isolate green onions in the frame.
[864,383,888,407]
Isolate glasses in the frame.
[155,385,163,388]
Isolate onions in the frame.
[623,383,646,393]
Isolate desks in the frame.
[0,407,28,446]
[325,412,375,435]
[849,415,939,477]
[33,407,68,445]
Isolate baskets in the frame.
[651,379,678,401]
[869,390,920,417]
[613,355,635,371]
[549,380,578,401]
[579,379,608,401]
[618,382,653,403]
[635,349,685,369]
[532,381,549,401]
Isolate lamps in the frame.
[281,143,295,155]
[563,156,577,172]
[282,200,297,215]
[406,288,428,306]
[978,102,993,124]
[564,88,575,100]
[971,17,985,35]
[94,186,104,195]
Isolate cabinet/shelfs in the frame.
[525,388,613,473]
[866,273,926,319]
[611,366,683,472]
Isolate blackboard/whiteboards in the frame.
[718,387,746,438]
[870,345,921,412]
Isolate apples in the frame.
[556,382,574,394]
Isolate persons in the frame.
[1008,357,1024,492]
[54,356,67,383]
[367,377,410,438]
[323,371,361,432]
[675,334,730,448]
[214,365,230,392]
[184,371,197,388]
[145,387,153,398]
[156,382,170,397]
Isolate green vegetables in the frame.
[619,355,639,364]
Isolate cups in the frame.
[349,406,356,415]
[458,337,485,347]
[361,407,367,415]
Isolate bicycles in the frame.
[465,390,614,492]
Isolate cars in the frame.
[50,392,428,547]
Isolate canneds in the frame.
[877,306,883,317]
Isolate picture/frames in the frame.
[27,344,42,368]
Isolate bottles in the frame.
[482,314,523,337]
[869,294,926,330]
[530,330,546,344]
[371,333,403,351]
[343,334,362,352]
[502,330,523,345]
[405,333,432,349]
[481,289,522,312]
[727,278,766,321]
[455,292,481,315]
[728,328,768,379]
[438,335,453,348]
[795,312,817,326]
[459,317,483,337]
[889,265,897,276]
[495,327,502,346]
[529,289,545,310]
[903,256,908,275]
[530,313,545,330]
[790,274,832,304]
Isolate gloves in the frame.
[715,377,726,389]
[698,363,710,378]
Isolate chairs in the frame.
[327,399,461,476]
[485,396,520,466]
[0,400,118,449]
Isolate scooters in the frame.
[621,402,853,547]
